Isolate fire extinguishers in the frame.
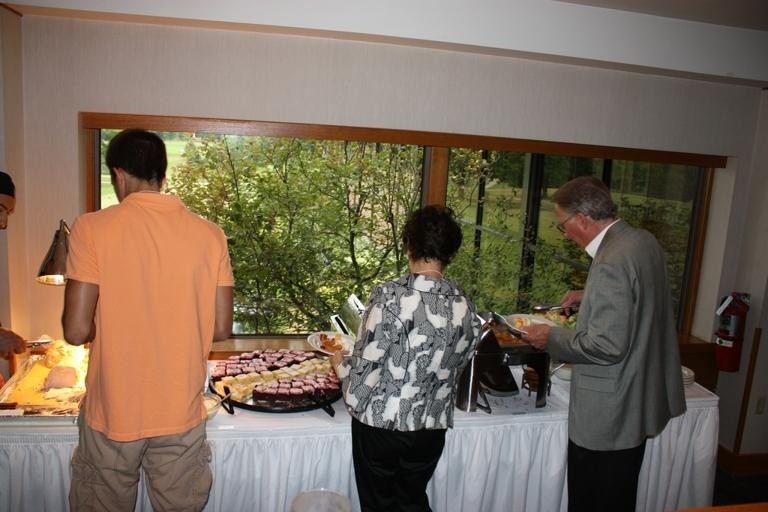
[713,290,750,372]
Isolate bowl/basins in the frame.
[201,392,221,419]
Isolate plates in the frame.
[307,330,357,355]
[24,340,52,348]
[495,311,559,336]
[679,363,695,389]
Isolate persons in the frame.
[519,174,687,511]
[61,128,237,512]
[330,202,484,512]
[0,167,29,367]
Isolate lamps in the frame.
[35,220,73,287]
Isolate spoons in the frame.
[531,303,576,313]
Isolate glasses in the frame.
[557,211,579,233]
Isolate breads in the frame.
[494,317,530,345]
[209,349,342,404]
[30,334,90,403]
